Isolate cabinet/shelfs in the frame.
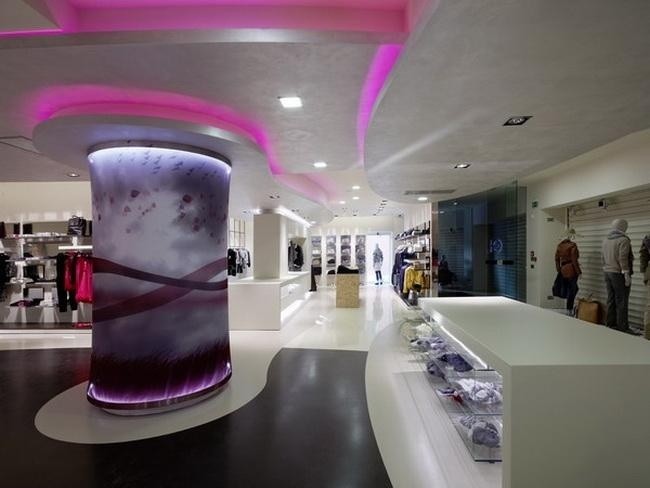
[413,293,648,485]
[1,220,91,330]
[389,220,431,312]
[227,232,365,330]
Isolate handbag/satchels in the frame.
[561,262,577,280]
[576,296,606,325]
[67,215,92,236]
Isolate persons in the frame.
[600,218,635,335]
[554,227,584,318]
[372,242,383,285]
[439,254,449,288]
[639,234,650,340]
[288,239,305,272]
